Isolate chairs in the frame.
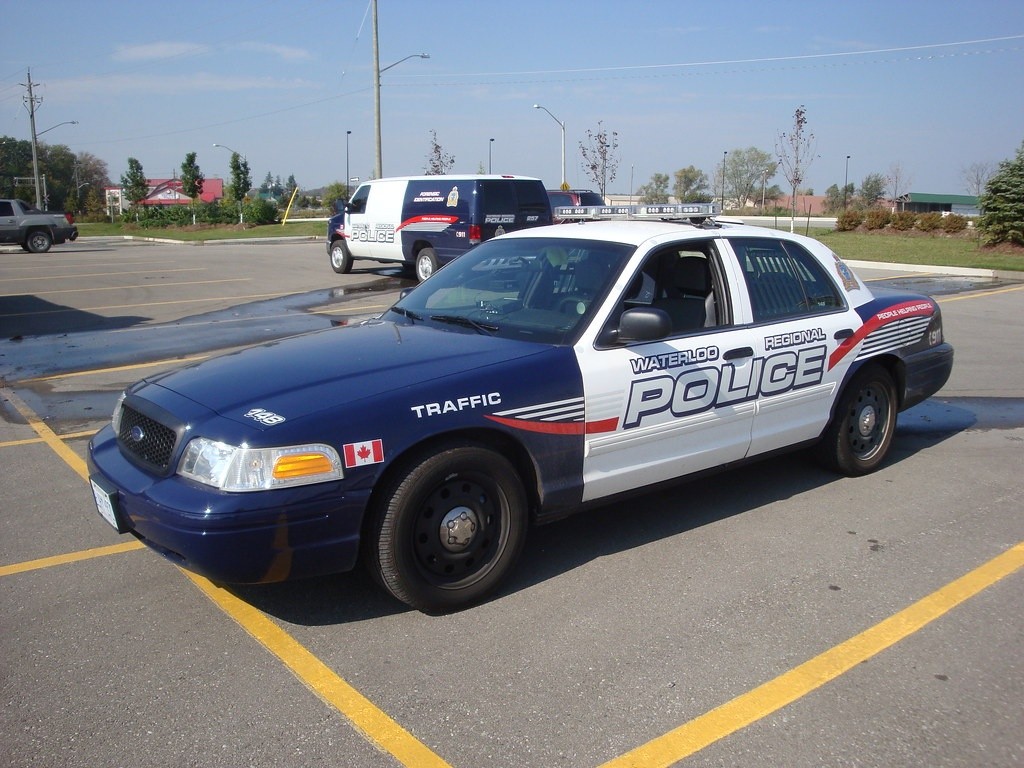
[538,258,624,321]
[651,256,714,332]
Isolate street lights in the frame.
[32,120,79,210]
[845,155,850,211]
[374,53,430,179]
[534,103,565,191]
[721,151,727,210]
[488,139,495,175]
[346,131,352,196]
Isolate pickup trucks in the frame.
[0,198,78,253]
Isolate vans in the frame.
[326,174,553,283]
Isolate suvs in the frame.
[545,189,611,225]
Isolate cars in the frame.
[86,204,954,613]
